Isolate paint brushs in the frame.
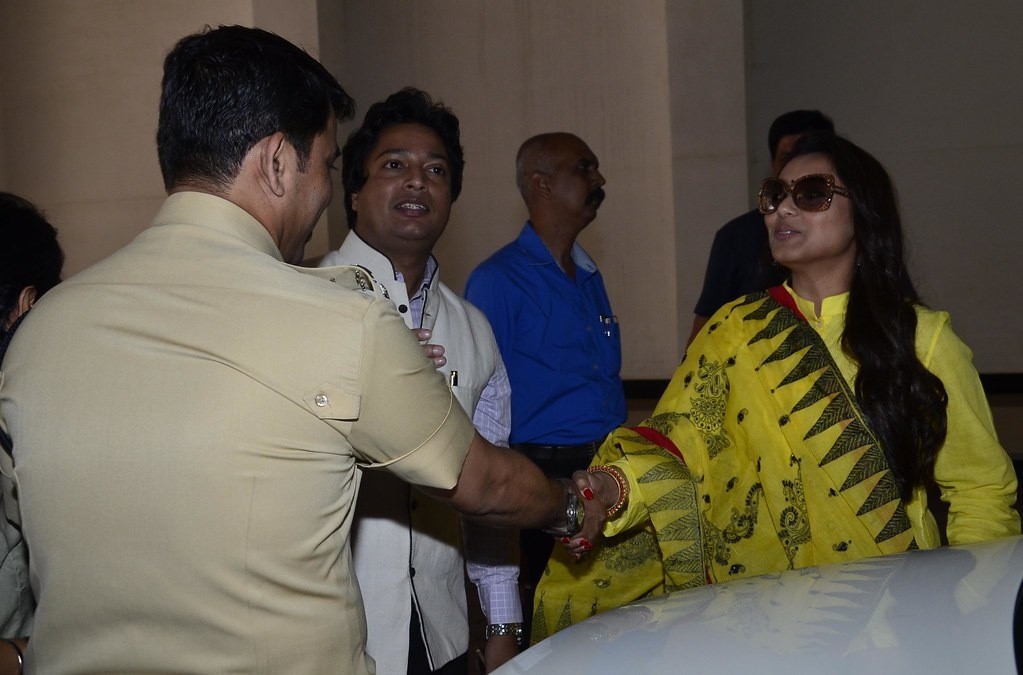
[449,370,458,386]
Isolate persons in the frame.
[0,191,68,675]
[301,84,526,674]
[460,127,630,618]
[682,109,841,356]
[528,129,1023,650]
[1,18,606,673]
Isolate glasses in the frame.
[759,174,848,214]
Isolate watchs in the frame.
[483,621,526,648]
[541,477,582,536]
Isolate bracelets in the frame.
[583,463,628,518]
[2,638,25,675]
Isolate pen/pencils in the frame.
[599,315,618,337]
